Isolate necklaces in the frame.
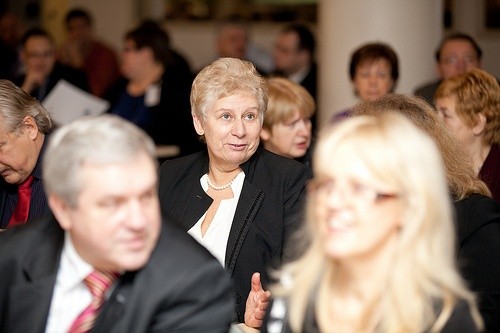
[206,173,235,190]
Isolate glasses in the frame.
[306,182,398,203]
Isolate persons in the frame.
[0,7,500,333]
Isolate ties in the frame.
[7,176,33,229]
[68,269,118,333]
